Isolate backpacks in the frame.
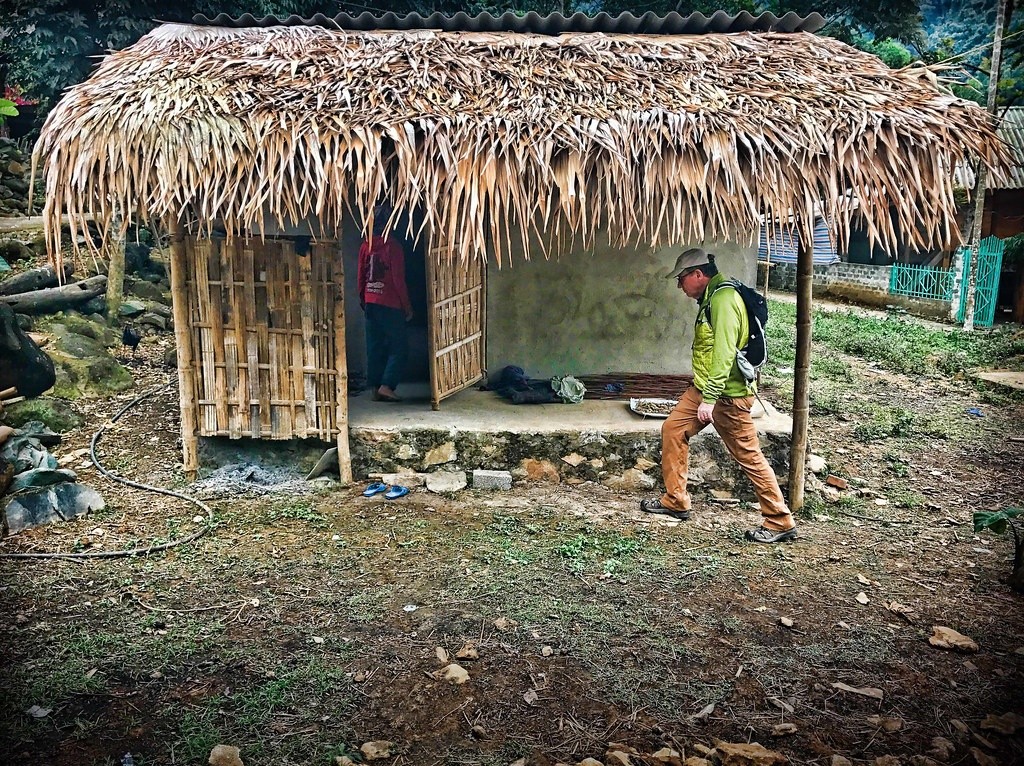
[706,276,768,383]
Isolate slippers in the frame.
[386,485,408,499]
[363,483,387,497]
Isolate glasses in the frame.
[675,270,695,281]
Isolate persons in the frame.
[357,208,412,403]
[639,249,797,544]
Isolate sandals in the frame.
[641,496,690,520]
[745,525,797,542]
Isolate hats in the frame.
[665,248,715,279]
